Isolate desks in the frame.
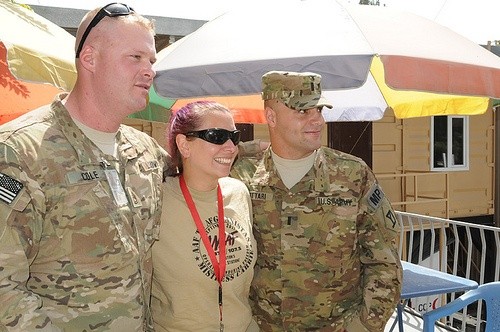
[388,259,479,332]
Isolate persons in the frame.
[229,71,403,332]
[0,3,271,332]
[149,103,260,332]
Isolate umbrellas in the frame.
[148,0,500,121]
[0,0,77,128]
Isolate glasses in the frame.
[75,3,135,58]
[181,128,241,146]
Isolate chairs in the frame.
[422,282,500,332]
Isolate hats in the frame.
[261,70,334,109]
